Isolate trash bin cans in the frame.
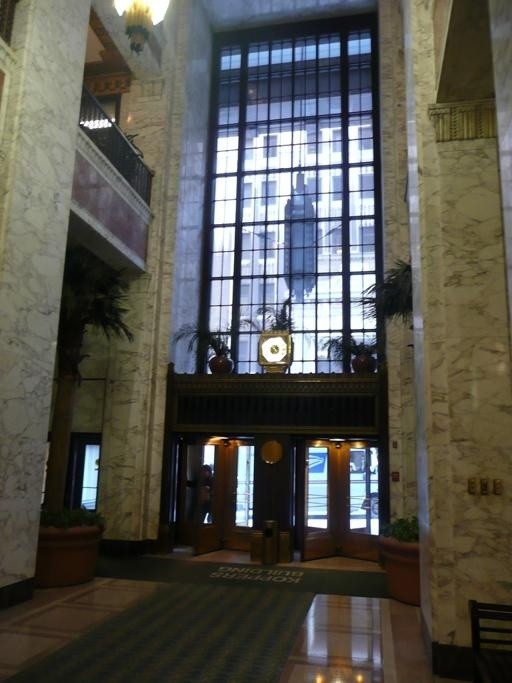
[262,520,279,564]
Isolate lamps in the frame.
[114,2,170,53]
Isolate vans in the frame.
[308,448,379,519]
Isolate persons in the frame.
[186,465,215,525]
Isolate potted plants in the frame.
[379,516,420,607]
[320,333,378,375]
[170,324,239,375]
[36,505,106,589]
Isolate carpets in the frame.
[1,583,315,682]
[95,555,387,600]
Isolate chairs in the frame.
[469,599,512,683]
